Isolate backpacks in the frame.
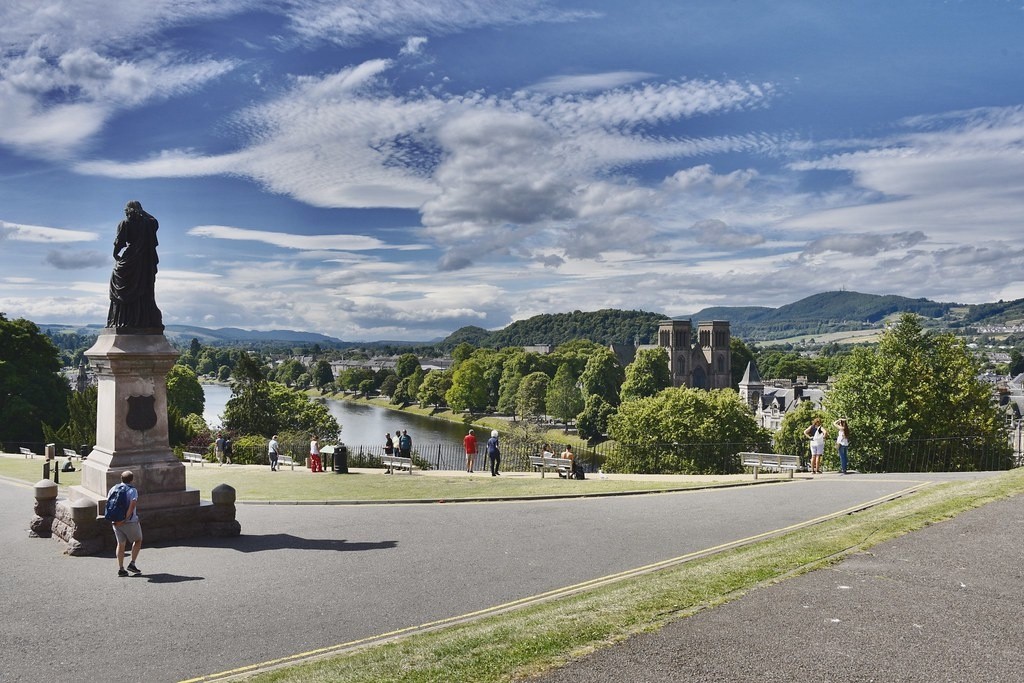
[105,485,136,521]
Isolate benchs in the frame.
[277,454,300,470]
[380,454,420,475]
[529,456,573,479]
[740,452,801,479]
[182,452,209,466]
[63,448,82,461]
[20,447,36,459]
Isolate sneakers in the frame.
[127,563,142,574]
[118,569,128,577]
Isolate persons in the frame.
[105,201,162,329]
[269,435,281,471]
[382,429,412,474]
[310,436,325,472]
[107,469,142,576]
[61,455,76,472]
[540,443,578,479]
[803,418,851,474]
[214,432,232,467]
[462,428,501,476]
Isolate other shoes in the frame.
[384,467,409,474]
[272,468,276,471]
[492,471,500,476]
[467,469,474,473]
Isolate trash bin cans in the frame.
[80,445,88,457]
[334,446,348,474]
